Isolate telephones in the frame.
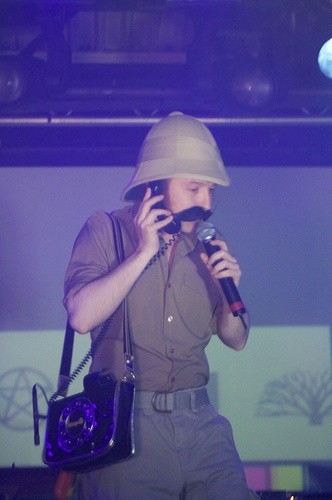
[148,181,180,234]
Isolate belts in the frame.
[136,388,210,414]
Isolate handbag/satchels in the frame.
[41,370,136,473]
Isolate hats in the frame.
[121,111,230,202]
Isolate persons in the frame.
[63,112,250,500]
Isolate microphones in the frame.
[196,222,249,329]
[32,385,40,444]
